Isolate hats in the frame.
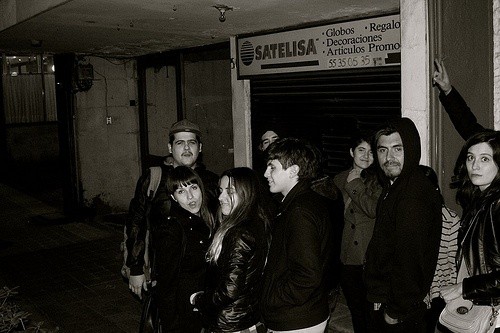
[168,119,201,137]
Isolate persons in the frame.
[431,58,485,142]
[362,118,442,333]
[190,167,272,333]
[334,136,383,333]
[125,120,220,333]
[257,137,332,333]
[419,164,462,333]
[258,129,279,152]
[294,139,344,333]
[154,166,220,333]
[439,128,500,333]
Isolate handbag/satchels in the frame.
[119,166,161,284]
[139,290,162,333]
[438,294,499,333]
